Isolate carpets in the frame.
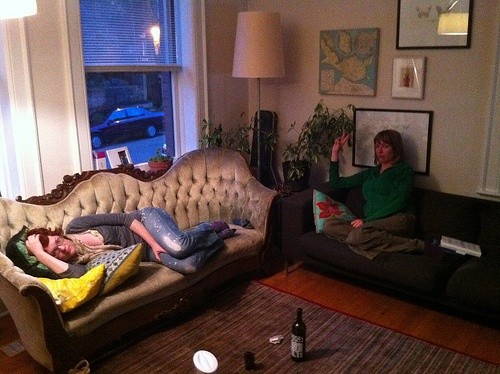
[97,279,500,374]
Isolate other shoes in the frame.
[65,359,91,374]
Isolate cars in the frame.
[89,107,166,150]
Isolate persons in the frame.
[25,208,237,278]
[324,129,446,260]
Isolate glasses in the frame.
[50,236,64,257]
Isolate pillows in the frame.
[86,242,144,296]
[314,188,359,234]
[6,224,56,279]
[38,263,105,313]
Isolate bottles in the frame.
[291,307,306,360]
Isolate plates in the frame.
[192,348,219,373]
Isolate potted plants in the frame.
[282,99,356,190]
[199,111,279,178]
[148,148,174,172]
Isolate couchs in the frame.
[282,178,500,332]
[0,145,283,374]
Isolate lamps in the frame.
[232,11,288,180]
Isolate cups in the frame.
[244,351,255,370]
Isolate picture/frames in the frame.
[352,107,434,177]
[396,0,474,50]
